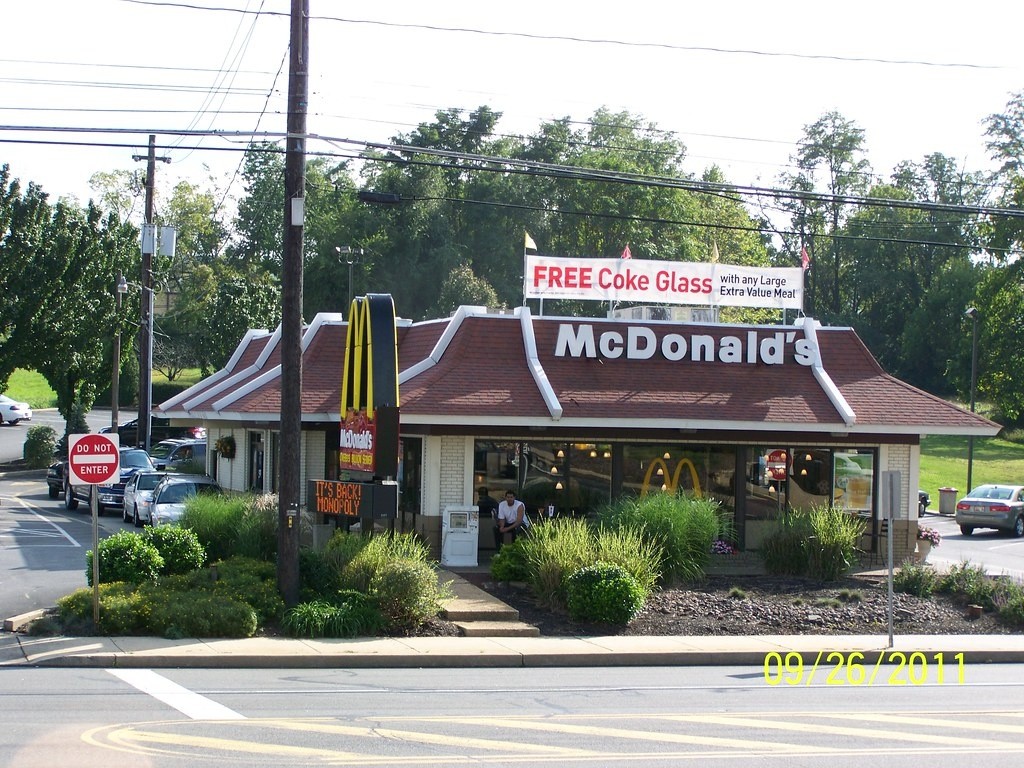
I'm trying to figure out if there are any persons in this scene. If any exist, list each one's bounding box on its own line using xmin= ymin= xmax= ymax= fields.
xmin=494 ymin=490 xmax=531 ymax=552
xmin=477 ymin=487 xmax=499 ymax=518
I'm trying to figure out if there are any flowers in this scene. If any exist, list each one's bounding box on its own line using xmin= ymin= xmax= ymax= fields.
xmin=916 ymin=524 xmax=941 ymax=549
xmin=214 ymin=436 xmax=235 ymax=453
xmin=710 ymin=540 xmax=733 ymax=557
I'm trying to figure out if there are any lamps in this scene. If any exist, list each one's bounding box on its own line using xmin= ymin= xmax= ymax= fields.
xmin=551 ymin=466 xmax=558 ymax=473
xmin=555 ymin=481 xmax=563 ymax=490
xmin=806 ymin=449 xmax=812 ymax=460
xmin=657 ymin=467 xmax=664 ymax=475
xmin=556 ymin=450 xmax=565 ymax=457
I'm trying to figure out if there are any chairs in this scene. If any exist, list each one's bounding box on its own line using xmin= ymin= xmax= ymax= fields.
xmin=861 ymin=518 xmax=894 ymax=569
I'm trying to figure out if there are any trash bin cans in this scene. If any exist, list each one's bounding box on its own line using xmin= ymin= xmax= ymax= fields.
xmin=938 ymin=486 xmax=958 ymax=514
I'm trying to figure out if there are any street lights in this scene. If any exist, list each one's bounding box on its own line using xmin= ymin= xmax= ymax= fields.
xmin=965 ymin=308 xmax=978 ymax=496
xmin=116 ymin=276 xmax=155 ymax=452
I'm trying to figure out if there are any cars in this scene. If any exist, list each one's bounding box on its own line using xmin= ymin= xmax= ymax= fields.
xmin=919 ymin=490 xmax=932 ymax=518
xmin=0 ymin=394 xmax=33 ymax=425
xmin=46 ymin=453 xmax=69 ymax=498
xmin=956 ymin=484 xmax=1024 ymax=538
xmin=148 ymin=437 xmax=206 ymax=474
xmin=145 ymin=472 xmax=228 ymax=531
xmin=62 ymin=447 xmax=165 ymax=516
xmin=122 ymin=469 xmax=185 ymax=527
xmin=97 ymin=412 xmax=206 ymax=452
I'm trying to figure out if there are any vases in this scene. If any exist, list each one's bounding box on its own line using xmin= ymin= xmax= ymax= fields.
xmin=222 ymin=454 xmax=234 ymax=458
xmin=916 ymin=539 xmax=933 ymax=565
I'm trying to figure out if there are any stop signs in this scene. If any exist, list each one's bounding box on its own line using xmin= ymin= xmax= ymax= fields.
xmin=70 ymin=435 xmax=119 ymax=483
xmin=768 ymin=450 xmax=793 ymax=474
